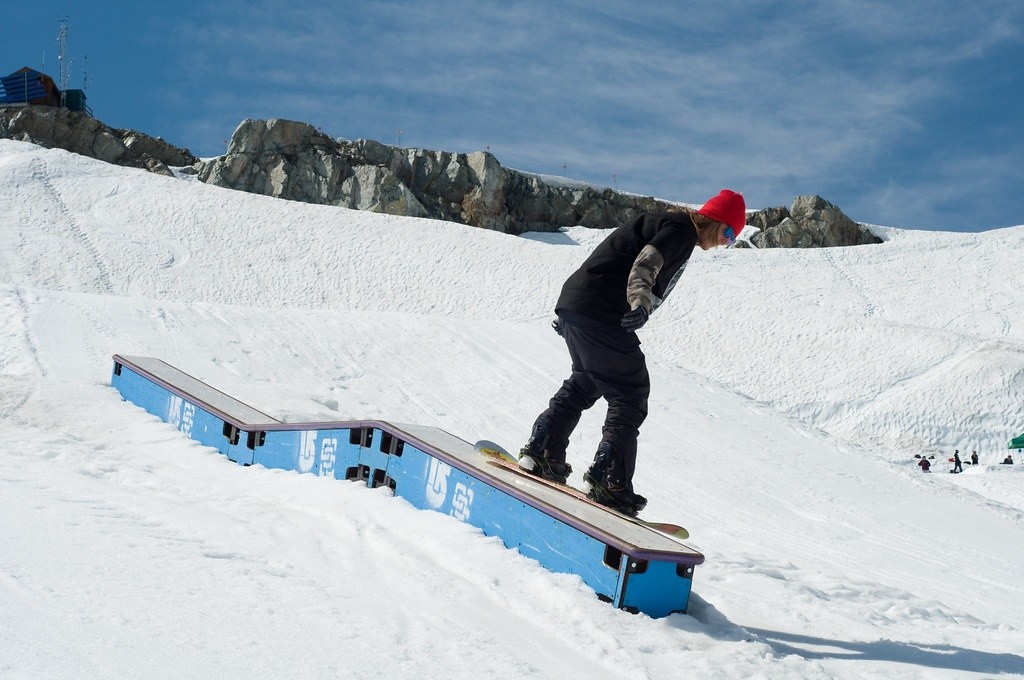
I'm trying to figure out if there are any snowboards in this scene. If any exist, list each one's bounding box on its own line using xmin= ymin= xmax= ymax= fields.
xmin=473 ymin=438 xmax=692 ymax=541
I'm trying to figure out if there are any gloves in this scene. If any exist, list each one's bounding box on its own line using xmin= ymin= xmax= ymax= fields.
xmin=621 ymin=306 xmax=651 ymax=332
xmin=552 ymin=315 xmax=566 ymax=337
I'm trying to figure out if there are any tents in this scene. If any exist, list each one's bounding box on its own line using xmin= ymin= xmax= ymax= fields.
xmin=1007 ymin=433 xmax=1024 ymax=455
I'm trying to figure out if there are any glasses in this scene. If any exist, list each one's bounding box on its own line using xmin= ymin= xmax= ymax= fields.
xmin=723 ymin=222 xmax=736 ymax=241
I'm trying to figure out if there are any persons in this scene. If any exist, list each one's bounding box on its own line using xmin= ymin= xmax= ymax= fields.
xmin=971 ymin=451 xmax=979 ymax=465
xmin=918 ymin=456 xmax=931 ymax=473
xmin=953 ymin=449 xmax=963 ymax=473
xmin=518 ymin=190 xmax=746 ymax=517
xmin=1004 ymin=455 xmax=1013 ymax=464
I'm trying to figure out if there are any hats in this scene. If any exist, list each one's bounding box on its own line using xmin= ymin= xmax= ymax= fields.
xmin=697 ymin=190 xmax=746 ymax=239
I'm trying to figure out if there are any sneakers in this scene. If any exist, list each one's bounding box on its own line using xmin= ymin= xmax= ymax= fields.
xmin=583 ymin=438 xmax=648 ymax=512
xmin=515 ymin=413 xmax=572 ymax=484
